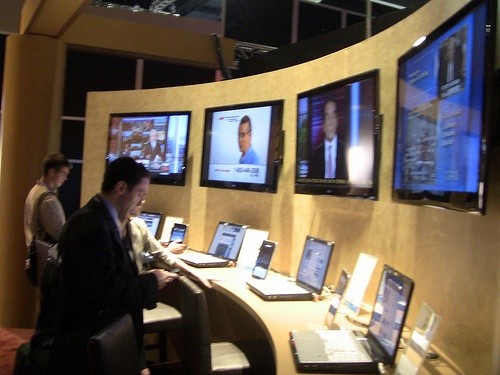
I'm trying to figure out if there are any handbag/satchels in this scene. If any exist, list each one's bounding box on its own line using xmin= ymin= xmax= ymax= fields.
xmin=26 ymin=234 xmax=59 ymax=286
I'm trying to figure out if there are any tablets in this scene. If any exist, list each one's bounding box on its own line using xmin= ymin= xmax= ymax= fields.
xmin=251 ymin=240 xmax=276 ymax=280
xmin=168 ymin=223 xmax=187 ymax=247
xmin=324 ymin=270 xmax=349 ymax=329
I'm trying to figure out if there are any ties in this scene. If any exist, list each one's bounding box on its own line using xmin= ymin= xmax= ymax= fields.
xmin=328 ymin=145 xmax=332 ymax=178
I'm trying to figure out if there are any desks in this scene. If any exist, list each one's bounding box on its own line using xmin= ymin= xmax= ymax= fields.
xmin=140 ymin=244 xmax=465 ymax=375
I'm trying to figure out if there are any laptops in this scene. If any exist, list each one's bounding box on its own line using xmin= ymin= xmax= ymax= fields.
xmin=135 ymin=211 xmax=163 ymax=239
xmin=246 ymin=235 xmax=336 ymax=302
xmin=175 ymin=221 xmax=248 ymax=268
xmin=289 ymin=264 xmax=415 ymax=373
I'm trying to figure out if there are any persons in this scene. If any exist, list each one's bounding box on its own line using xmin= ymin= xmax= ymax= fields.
xmin=57 ymin=156 xmax=179 ymax=375
xmin=238 ymin=116 xmax=259 ymax=164
xmin=23 ymin=152 xmax=73 ymax=325
xmin=438 ymin=36 xmax=464 ymax=95
xmin=144 ymin=129 xmax=161 ymax=161
xmin=306 ymin=98 xmax=348 ymax=179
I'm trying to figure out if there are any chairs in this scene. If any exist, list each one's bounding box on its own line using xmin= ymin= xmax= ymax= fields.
xmin=33 ymin=237 xmax=55 ymax=331
xmin=85 ymin=311 xmax=142 ymax=375
xmin=140 ymin=301 xmax=183 ymax=375
xmin=172 ymin=275 xmax=251 ymax=375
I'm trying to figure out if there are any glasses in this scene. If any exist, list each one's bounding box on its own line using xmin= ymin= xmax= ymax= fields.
xmin=239 ymin=131 xmax=249 ymax=137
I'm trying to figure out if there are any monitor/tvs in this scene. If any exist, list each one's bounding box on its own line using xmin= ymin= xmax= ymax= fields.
xmin=105 ymin=110 xmax=192 ymax=186
xmin=200 ymin=99 xmax=284 ymax=194
xmin=294 ymin=68 xmax=381 ymax=200
xmin=392 ymin=0 xmax=498 ymax=215
